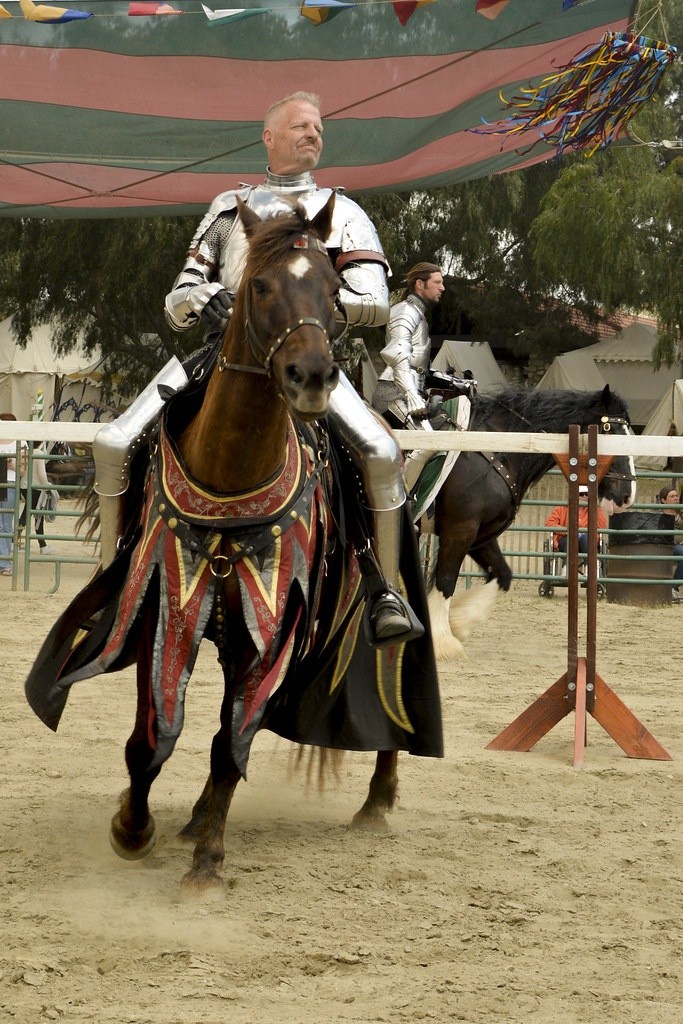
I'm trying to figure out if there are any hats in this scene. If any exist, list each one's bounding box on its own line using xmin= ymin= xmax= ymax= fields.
xmin=579 ymin=485 xmax=588 ymax=492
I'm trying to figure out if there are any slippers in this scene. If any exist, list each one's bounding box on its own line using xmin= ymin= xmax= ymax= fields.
xmin=1 ymin=568 xmax=12 ymax=576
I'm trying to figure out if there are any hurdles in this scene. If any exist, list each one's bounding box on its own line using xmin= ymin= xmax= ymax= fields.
xmin=0 ymin=418 xmax=682 ymax=766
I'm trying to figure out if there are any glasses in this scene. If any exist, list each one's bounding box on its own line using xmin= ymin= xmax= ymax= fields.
xmin=579 ymin=492 xmax=588 ymax=496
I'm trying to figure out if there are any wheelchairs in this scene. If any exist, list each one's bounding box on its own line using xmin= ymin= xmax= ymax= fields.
xmin=537 ymin=531 xmax=605 ymax=601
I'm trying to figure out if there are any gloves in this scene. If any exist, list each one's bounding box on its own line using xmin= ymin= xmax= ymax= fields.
xmin=393 ymin=369 xmax=429 ymax=422
xmin=424 ymin=367 xmax=478 ymax=394
xmin=164 ymin=280 xmax=233 ymax=328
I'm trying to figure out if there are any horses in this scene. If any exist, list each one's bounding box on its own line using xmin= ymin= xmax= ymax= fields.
xmin=416 ymin=382 xmax=637 ymax=599
xmin=106 ymin=194 xmax=401 ymax=880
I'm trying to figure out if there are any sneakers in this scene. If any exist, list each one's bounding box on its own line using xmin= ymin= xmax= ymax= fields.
xmin=672 ymin=587 xmax=681 ymax=599
xmin=40 ymin=543 xmax=57 ymax=555
xmin=561 ymin=565 xmax=566 ymax=577
xmin=373 ymin=598 xmax=412 ymax=639
xmin=583 ymin=564 xmax=587 ymax=576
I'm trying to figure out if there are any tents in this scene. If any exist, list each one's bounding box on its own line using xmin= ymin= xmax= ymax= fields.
xmin=529 ymin=353 xmax=607 ymax=393
xmin=355 ymin=338 xmax=379 ymax=404
xmin=0 ymin=309 xmax=168 ymax=424
xmin=563 ymin=322 xmax=683 ymax=425
xmin=431 ymin=340 xmax=510 ymax=395
xmin=633 ymin=379 xmax=683 ymax=471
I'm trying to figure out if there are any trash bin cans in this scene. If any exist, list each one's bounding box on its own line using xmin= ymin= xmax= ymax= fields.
xmin=608 ymin=512 xmax=675 ymax=609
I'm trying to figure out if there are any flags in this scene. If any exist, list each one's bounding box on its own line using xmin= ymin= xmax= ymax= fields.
xmin=20 ymin=0 xmax=94 ymax=24
xmin=0 ymin=4 xmax=11 ymax=19
xmin=201 ymin=2 xmax=272 ymax=28
xmin=392 ymin=0 xmax=437 ymax=27
xmin=127 ymin=2 xmax=183 ymax=15
xmin=475 ymin=0 xmax=511 ymax=20
xmin=300 ymin=0 xmax=356 ymax=26
xmin=562 ymin=0 xmax=593 ymax=11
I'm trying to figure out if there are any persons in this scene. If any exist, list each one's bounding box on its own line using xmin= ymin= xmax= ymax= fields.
xmin=90 ymin=92 xmax=415 ymax=638
xmin=372 ymin=262 xmax=477 ymax=496
xmin=0 ymin=413 xmax=52 ymax=575
xmin=545 ymin=485 xmax=608 ymax=577
xmin=657 ymin=487 xmax=683 ymax=598
